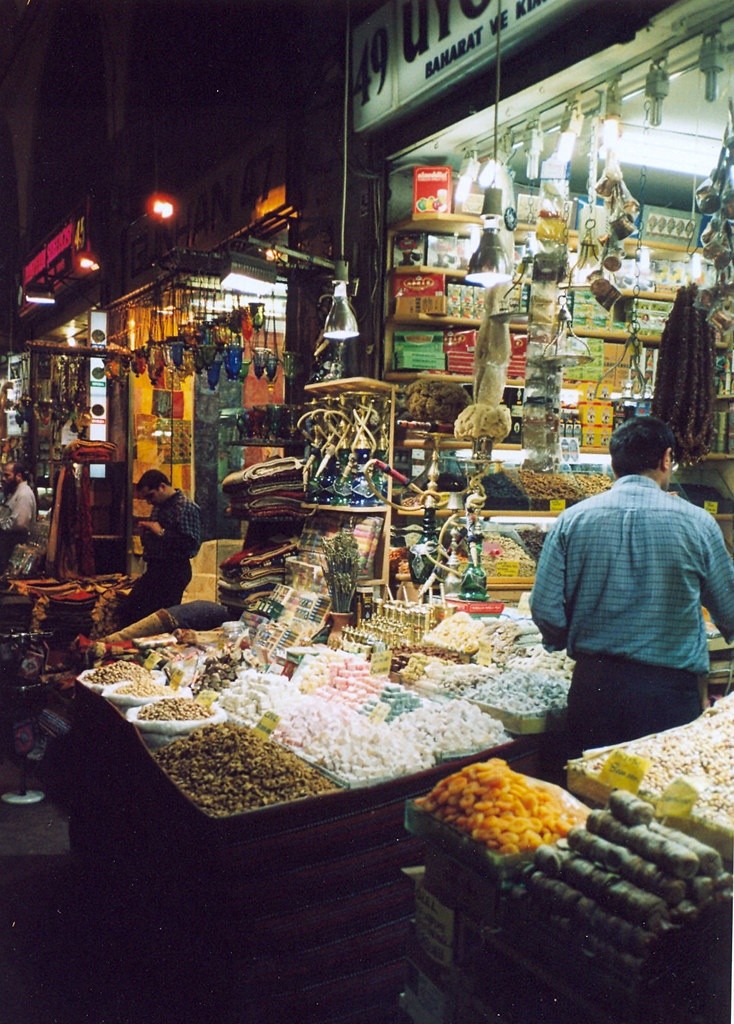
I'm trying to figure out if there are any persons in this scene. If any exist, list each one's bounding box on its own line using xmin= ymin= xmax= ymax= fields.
xmin=118 ymin=469 xmax=202 ymax=631
xmin=529 ymin=417 xmax=734 ymax=791
xmin=0 ymin=460 xmax=37 ymax=577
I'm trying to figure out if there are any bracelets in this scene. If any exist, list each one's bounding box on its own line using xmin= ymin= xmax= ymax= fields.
xmin=157 ymin=528 xmax=165 ymax=537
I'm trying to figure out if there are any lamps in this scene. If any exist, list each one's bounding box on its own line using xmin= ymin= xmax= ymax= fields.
xmin=464 ymin=0 xmax=514 ymax=288
xmin=220 ymin=238 xmax=276 ymax=295
xmin=25 ymin=281 xmax=55 ymax=304
xmin=323 ymin=0 xmax=360 ymax=339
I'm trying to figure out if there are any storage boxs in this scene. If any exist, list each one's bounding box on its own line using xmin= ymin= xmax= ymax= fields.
xmin=392 ymin=274 xmax=675 ymax=336
xmin=392 ymin=330 xmax=659 ymax=400
xmin=463 ymin=385 xmax=635 ymax=448
xmin=413 ymin=165 xmax=702 ymax=247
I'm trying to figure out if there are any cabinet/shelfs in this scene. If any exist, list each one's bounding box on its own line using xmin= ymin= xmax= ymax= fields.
xmin=219 ymin=439 xmax=310 ymax=609
xmin=383 ymin=212 xmax=734 ymax=601
xmin=300 ymin=377 xmax=395 ymax=602
xmin=54 ymin=459 xmax=129 ymax=539
